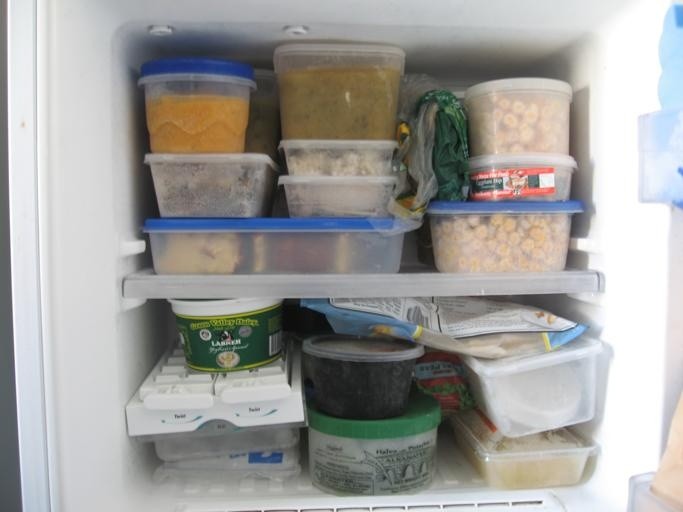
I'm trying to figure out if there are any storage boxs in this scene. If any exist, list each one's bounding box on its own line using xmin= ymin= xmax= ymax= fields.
xmin=140 ymin=216 xmax=409 ymax=274
